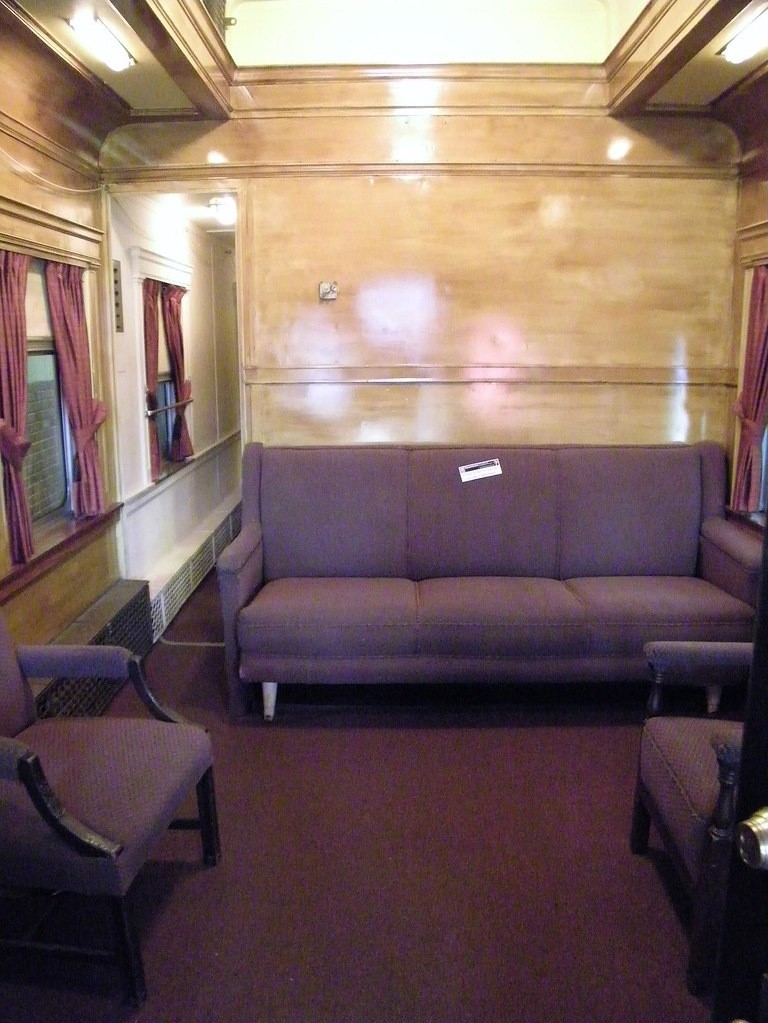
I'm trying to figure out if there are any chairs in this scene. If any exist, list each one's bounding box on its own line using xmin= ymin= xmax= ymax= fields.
xmin=629 ymin=642 xmax=756 ymax=993
xmin=0 ymin=608 xmax=218 ymax=1000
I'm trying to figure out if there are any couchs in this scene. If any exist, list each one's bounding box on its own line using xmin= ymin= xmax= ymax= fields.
xmin=213 ymin=443 xmax=768 ymax=721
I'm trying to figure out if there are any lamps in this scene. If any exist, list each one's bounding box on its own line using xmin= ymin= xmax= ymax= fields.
xmin=721 ymin=7 xmax=768 ymax=64
xmin=62 ymin=15 xmax=138 ymax=71
